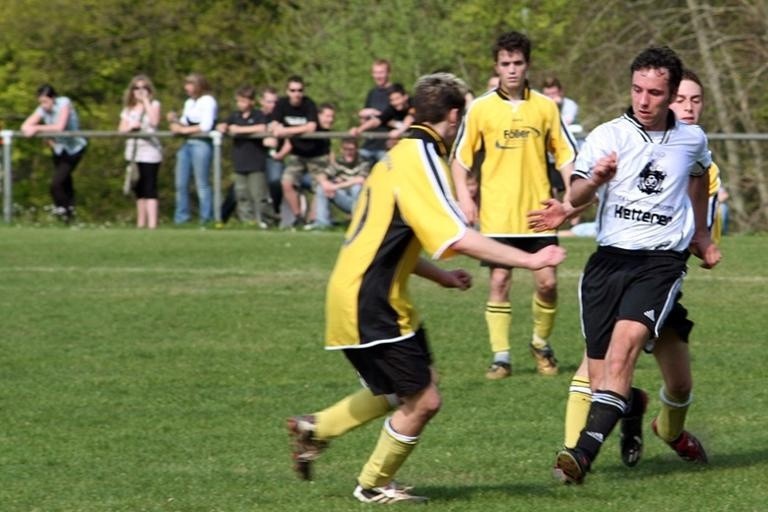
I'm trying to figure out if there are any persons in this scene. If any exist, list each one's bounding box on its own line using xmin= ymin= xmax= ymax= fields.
xmin=449 ymin=32 xmax=579 ymax=379
xmin=286 ymin=72 xmax=567 ymax=507
xmin=21 ymin=84 xmax=86 ymax=228
xmin=118 ymin=74 xmax=161 ymax=230
xmin=527 ymin=73 xmax=720 ymax=481
xmin=557 ymin=46 xmax=721 ymax=487
xmin=166 ymin=74 xmax=218 ymax=229
xmin=218 ymin=61 xmax=582 ymax=232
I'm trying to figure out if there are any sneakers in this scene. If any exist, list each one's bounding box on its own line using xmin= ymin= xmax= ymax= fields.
xmin=353 ymin=480 xmax=428 ymax=505
xmin=484 ymin=360 xmax=511 ymax=380
xmin=528 ymin=339 xmax=560 ymax=376
xmin=286 ymin=414 xmax=328 ymax=484
xmin=555 ymin=387 xmax=707 ymax=486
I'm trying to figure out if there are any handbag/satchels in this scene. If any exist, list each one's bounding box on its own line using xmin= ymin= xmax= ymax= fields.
xmin=130 ymin=162 xmax=140 ymax=186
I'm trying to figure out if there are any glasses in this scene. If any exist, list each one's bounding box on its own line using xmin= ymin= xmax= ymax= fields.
xmin=289 ymin=87 xmax=304 ymax=94
